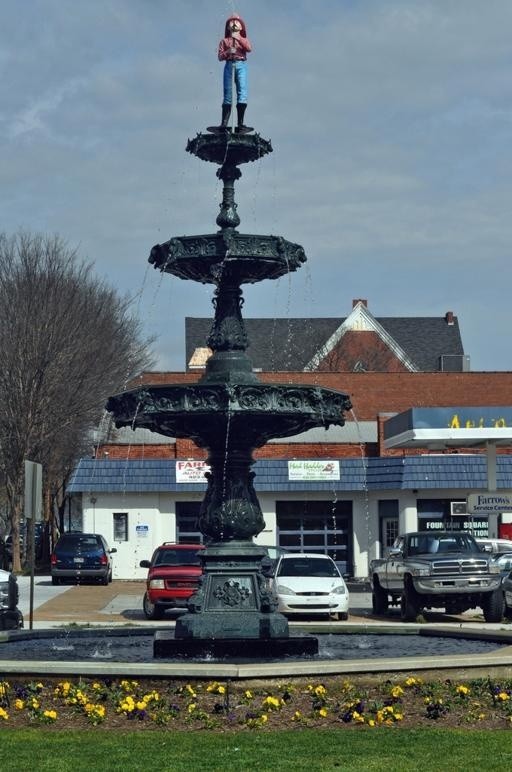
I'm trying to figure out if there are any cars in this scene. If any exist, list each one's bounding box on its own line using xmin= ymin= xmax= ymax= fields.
xmin=0 ymin=521 xmax=44 ymax=630
xmin=475 ymin=531 xmax=512 ymax=617
xmin=265 ymin=553 xmax=349 ymax=621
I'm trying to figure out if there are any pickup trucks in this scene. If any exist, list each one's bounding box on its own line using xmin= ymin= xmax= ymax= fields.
xmin=369 ymin=531 xmax=502 ymax=622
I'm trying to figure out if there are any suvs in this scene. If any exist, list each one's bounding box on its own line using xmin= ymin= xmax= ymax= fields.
xmin=140 ymin=541 xmax=206 ymax=620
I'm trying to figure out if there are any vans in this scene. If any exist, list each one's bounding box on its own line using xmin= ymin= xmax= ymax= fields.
xmin=51 ymin=533 xmax=117 ymax=585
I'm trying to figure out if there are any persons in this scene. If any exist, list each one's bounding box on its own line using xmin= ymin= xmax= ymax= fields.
xmin=217 ymin=15 xmax=255 ymax=134
